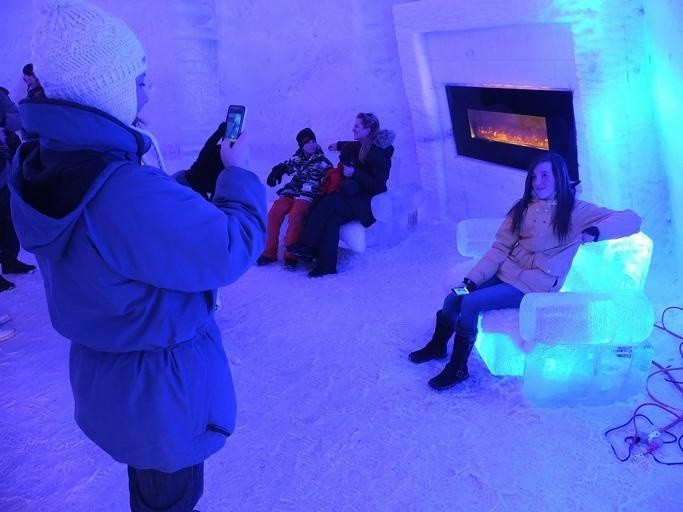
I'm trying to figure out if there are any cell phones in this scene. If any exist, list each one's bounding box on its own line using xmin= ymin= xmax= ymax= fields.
xmin=217 ymin=104 xmax=248 ymax=169
xmin=451 ymin=287 xmax=470 ymax=296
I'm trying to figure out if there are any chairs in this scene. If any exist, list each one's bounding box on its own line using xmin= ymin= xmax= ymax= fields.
xmin=444 ymin=215 xmax=654 ymax=409
xmin=337 ymin=158 xmax=422 ymax=256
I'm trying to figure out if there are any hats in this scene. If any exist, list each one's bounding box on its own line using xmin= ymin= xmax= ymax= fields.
xmin=30 ymin=0 xmax=147 ymax=125
xmin=296 ymin=128 xmax=317 ymax=150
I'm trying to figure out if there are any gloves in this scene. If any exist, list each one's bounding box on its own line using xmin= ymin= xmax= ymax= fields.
xmin=185 ymin=123 xmax=231 ymax=194
xmin=266 ymin=162 xmax=288 ymax=186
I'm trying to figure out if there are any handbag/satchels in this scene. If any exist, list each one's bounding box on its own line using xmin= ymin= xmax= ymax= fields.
xmin=320 ymin=162 xmax=342 ymax=197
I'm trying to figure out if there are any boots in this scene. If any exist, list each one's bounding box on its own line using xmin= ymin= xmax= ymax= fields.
xmin=427 ymin=328 xmax=477 ymax=390
xmin=408 ymin=308 xmax=456 ymax=364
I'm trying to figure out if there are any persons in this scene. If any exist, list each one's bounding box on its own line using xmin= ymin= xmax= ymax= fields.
xmin=408 ymin=154 xmax=641 ymax=391
xmin=0 ymin=61 xmax=45 ymax=342
xmin=255 ymin=112 xmax=395 ymax=277
xmin=11 ymin=4 xmax=268 ymax=512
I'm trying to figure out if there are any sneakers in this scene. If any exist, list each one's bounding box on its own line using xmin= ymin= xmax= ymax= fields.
xmin=256 ymin=240 xmax=338 ymax=278
xmin=1 ymin=259 xmax=37 ymax=293
